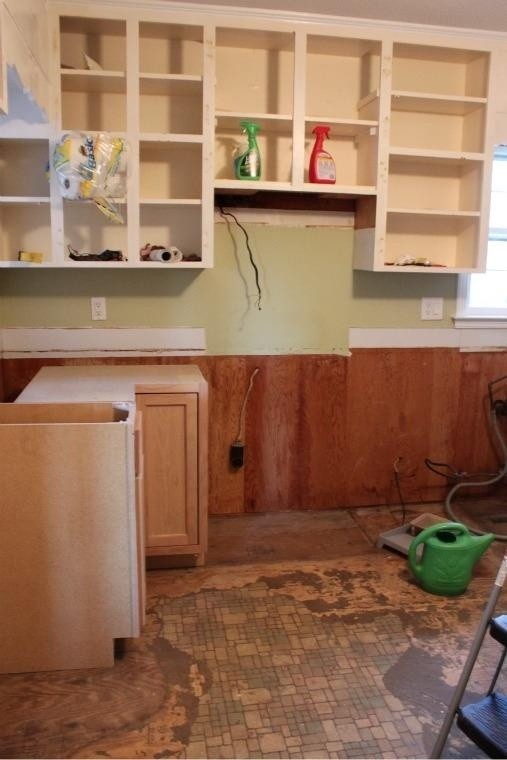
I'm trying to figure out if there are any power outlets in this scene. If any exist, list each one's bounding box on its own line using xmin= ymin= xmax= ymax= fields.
xmin=88 ymin=297 xmax=106 ymax=321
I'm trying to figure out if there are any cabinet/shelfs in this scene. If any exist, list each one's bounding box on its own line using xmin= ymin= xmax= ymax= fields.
xmin=8 ymin=362 xmax=218 ymax=577
xmin=0 ymin=3 xmax=506 ymax=277
xmin=0 ymin=399 xmax=146 ymax=683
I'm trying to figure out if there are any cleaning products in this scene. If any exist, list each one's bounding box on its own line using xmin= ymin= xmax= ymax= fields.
xmin=310 ymin=125 xmax=338 ymax=184
xmin=234 ymin=120 xmax=261 ymax=180
xmin=148 ymin=247 xmax=173 ymax=261
